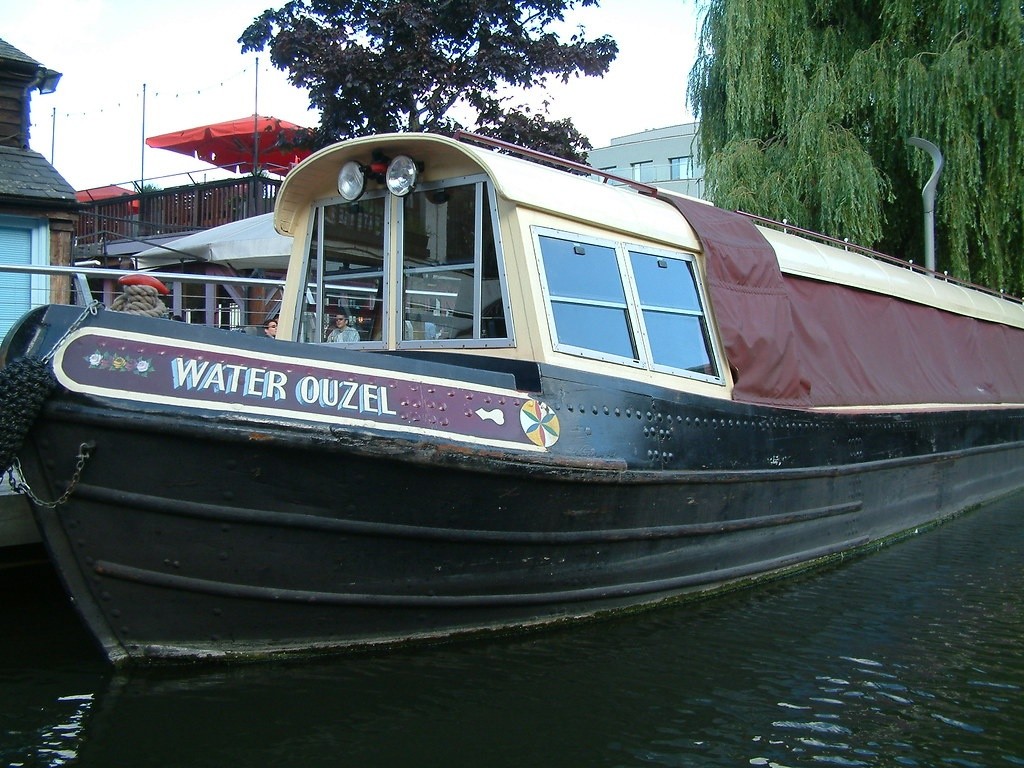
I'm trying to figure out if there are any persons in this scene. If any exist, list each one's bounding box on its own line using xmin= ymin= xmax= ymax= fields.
xmin=425 ymin=323 xmax=436 ymax=340
xmin=263 ymin=320 xmax=278 ymax=339
xmin=326 ymin=311 xmax=360 ymax=343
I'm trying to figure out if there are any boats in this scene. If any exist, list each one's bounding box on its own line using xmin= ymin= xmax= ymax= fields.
xmin=0 ymin=127 xmax=1024 ymax=675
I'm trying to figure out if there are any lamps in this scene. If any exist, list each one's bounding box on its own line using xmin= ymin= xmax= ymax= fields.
xmin=38 ymin=69 xmax=62 ymax=95
xmin=336 ymin=148 xmax=426 ymax=202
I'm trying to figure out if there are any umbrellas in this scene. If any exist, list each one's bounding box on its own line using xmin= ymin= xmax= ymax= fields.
xmin=76 ymin=184 xmax=139 ymax=216
xmin=145 ymin=113 xmax=317 ymax=178
